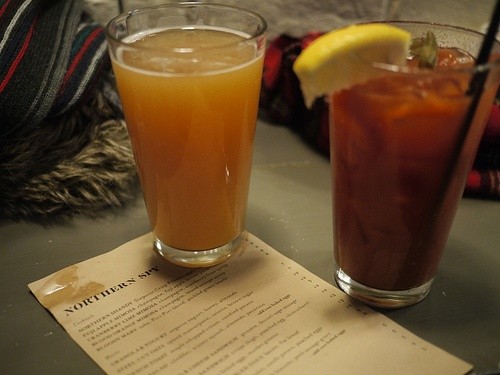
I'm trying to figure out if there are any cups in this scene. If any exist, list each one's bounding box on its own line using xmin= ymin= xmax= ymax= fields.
xmin=329 ymin=22 xmax=499 ymax=309
xmin=106 ymin=3 xmax=269 ymax=268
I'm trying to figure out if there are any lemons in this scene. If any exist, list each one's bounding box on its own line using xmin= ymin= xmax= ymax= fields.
xmin=293 ymin=23 xmax=412 ymax=107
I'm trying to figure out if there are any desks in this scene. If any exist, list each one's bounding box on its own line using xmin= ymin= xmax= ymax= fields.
xmin=0 ymin=117 xmax=500 ymax=375
